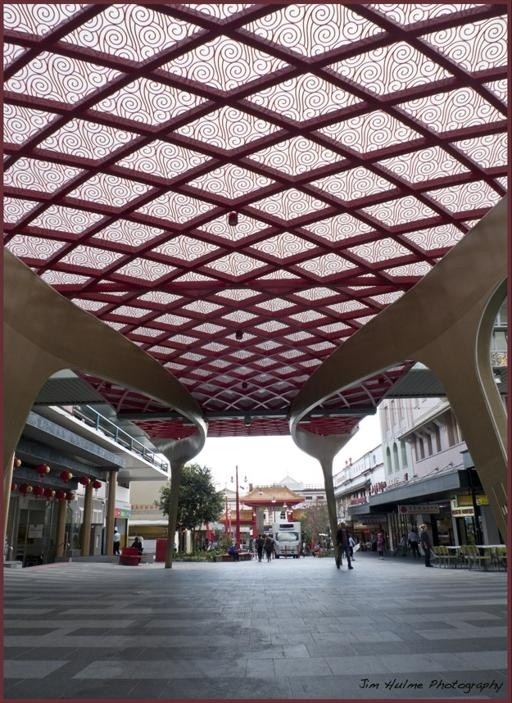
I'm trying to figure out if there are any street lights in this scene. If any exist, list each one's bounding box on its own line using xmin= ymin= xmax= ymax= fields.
xmin=227 ymin=509 xmax=232 ymax=539
xmin=460 ymin=449 xmax=488 ymax=571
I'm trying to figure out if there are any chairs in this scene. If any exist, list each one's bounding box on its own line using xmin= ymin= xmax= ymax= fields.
xmin=427 ymin=542 xmax=508 ymax=573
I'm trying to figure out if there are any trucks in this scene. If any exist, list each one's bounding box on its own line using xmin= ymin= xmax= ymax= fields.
xmin=273 ymin=521 xmax=301 ymax=559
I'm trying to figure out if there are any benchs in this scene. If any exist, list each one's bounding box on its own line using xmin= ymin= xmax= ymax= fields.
xmin=212 ymin=547 xmax=253 ymax=562
xmin=115 ymin=545 xmax=149 ymax=565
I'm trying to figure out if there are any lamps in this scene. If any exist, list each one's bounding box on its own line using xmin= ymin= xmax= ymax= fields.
xmin=224 ymin=209 xmax=252 ymax=428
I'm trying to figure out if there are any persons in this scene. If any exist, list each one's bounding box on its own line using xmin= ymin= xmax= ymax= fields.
xmin=417 ymin=522 xmax=433 ymax=567
xmin=114 ymin=525 xmax=419 ymax=561
xmin=336 ymin=521 xmax=354 ymax=569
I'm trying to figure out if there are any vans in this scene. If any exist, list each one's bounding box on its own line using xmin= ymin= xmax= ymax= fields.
xmin=318 ymin=532 xmax=331 ymax=547
